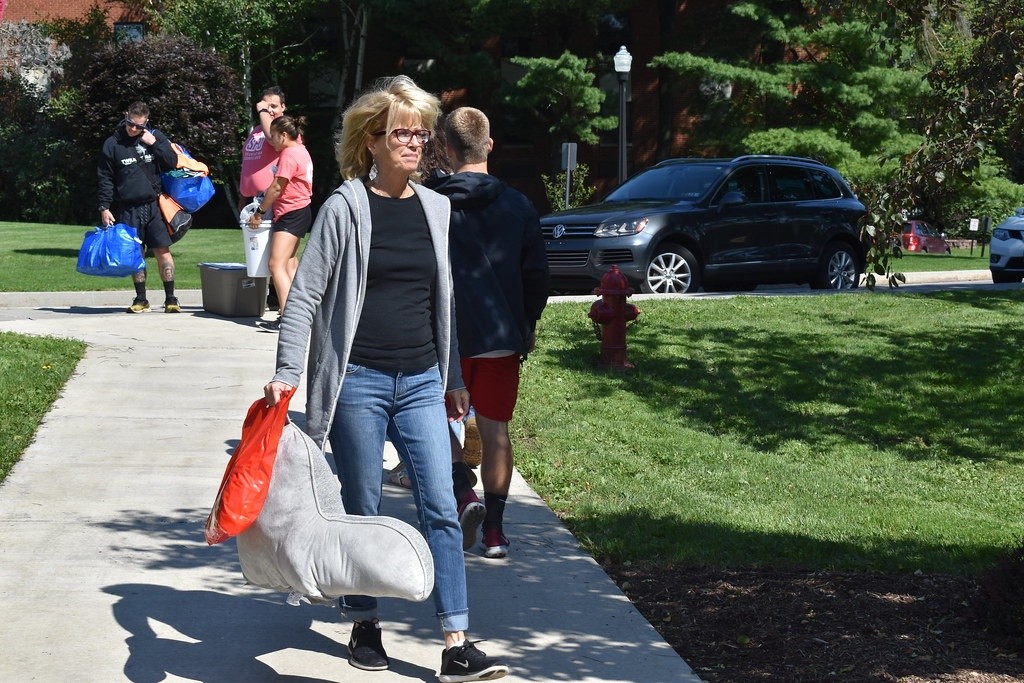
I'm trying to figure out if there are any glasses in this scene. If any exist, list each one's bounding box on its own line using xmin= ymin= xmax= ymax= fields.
xmin=125 ymin=113 xmax=148 ymax=130
xmin=372 ymin=127 xmax=431 ymax=144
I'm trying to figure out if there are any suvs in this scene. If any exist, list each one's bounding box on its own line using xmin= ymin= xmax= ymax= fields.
xmin=541 ymin=154 xmax=877 ymax=295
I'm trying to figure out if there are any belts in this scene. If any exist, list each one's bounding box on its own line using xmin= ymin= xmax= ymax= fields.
xmin=256 ymin=191 xmax=264 ymax=197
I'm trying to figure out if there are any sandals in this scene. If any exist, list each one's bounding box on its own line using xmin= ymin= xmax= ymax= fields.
xmin=389 ymin=471 xmax=413 ymax=488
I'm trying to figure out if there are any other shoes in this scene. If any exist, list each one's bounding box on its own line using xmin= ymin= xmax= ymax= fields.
xmin=264 ymin=293 xmax=281 ymax=310
xmin=463 ymin=463 xmax=478 ymax=486
xmin=464 ymin=415 xmax=485 ymax=467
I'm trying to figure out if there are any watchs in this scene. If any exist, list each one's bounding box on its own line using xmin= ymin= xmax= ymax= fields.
xmin=256 ymin=207 xmax=266 ymax=215
xmin=99 ymin=206 xmax=107 ymax=212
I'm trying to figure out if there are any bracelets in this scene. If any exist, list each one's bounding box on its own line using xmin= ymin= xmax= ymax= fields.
xmin=259 ymin=109 xmax=268 ymax=113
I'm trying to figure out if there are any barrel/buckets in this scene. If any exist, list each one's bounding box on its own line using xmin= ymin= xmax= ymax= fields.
xmin=239 ymin=220 xmax=274 ymax=277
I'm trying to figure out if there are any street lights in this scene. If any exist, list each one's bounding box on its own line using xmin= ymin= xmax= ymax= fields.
xmin=614 ymin=45 xmax=633 ymax=186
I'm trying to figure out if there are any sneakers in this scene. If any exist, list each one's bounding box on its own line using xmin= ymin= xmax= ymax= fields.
xmin=458 ymin=488 xmax=486 ymax=550
xmin=127 ymin=298 xmax=152 ymax=312
xmin=258 ymin=316 xmax=282 ymax=332
xmin=163 ymin=296 xmax=182 ymax=312
xmin=480 ymin=526 xmax=511 ymax=558
xmin=437 ymin=637 xmax=509 ymax=683
xmin=347 ymin=617 xmax=390 ymax=670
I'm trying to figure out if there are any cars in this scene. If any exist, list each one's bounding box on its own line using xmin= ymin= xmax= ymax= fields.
xmin=900 ymin=220 xmax=950 ymax=255
xmin=989 ymin=207 xmax=1024 ymax=283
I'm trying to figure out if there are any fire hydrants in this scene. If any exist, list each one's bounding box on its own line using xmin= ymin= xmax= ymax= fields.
xmin=588 ymin=264 xmax=642 ymax=370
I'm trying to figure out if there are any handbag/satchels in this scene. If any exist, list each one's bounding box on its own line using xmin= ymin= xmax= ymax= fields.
xmin=239 ymin=199 xmax=274 ymax=221
xmin=76 ymin=222 xmax=146 ymax=277
xmin=147 ymin=129 xmax=216 ymax=243
xmin=206 ymin=386 xmax=296 ymax=543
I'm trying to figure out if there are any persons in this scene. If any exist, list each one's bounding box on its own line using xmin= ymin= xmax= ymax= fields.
xmin=423 ymin=106 xmax=551 ymax=557
xmin=367 ymin=117 xmax=484 ymax=487
xmin=265 ymin=75 xmax=510 ymax=683
xmin=97 ymin=100 xmax=180 ymax=314
xmin=240 ymin=87 xmax=303 ymax=311
xmin=247 ymin=115 xmax=312 ymax=334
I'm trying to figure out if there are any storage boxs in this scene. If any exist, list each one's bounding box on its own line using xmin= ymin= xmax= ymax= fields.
xmin=198 ymin=262 xmax=268 ymax=318
